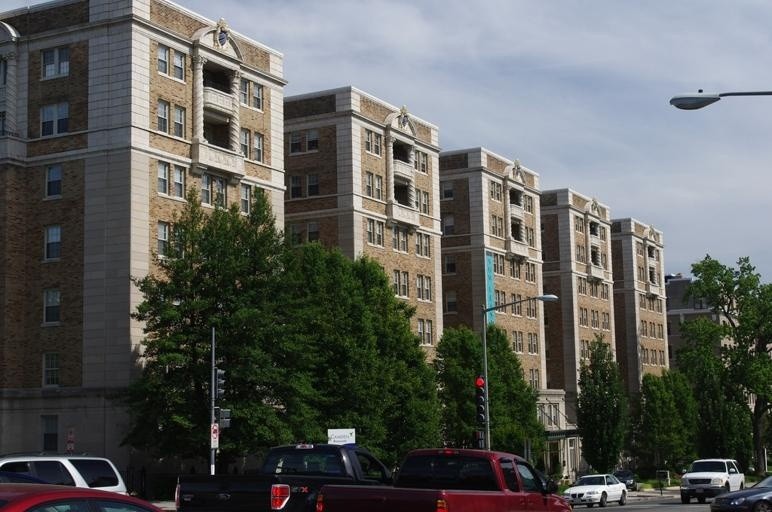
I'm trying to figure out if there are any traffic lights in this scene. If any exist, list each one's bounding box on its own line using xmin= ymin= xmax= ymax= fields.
xmin=473 ymin=372 xmax=486 ymax=418
xmin=213 ymin=366 xmax=226 ymax=400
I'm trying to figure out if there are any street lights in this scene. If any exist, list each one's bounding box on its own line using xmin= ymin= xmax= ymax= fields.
xmin=481 ymin=292 xmax=559 ymax=450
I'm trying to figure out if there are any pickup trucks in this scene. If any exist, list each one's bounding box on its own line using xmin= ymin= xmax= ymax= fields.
xmin=173 ymin=441 xmax=393 ymax=512
xmin=313 ymin=447 xmax=572 ymax=512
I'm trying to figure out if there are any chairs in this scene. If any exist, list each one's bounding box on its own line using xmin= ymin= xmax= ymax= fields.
xmin=324 ymin=457 xmax=339 ymax=471
xmin=287 ymin=455 xmax=309 ymax=474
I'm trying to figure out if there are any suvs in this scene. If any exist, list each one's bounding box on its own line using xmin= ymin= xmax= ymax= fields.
xmin=679 ymin=457 xmax=745 ymax=502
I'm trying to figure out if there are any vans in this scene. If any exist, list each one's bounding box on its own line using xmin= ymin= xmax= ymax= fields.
xmin=0 ymin=455 xmax=129 ymax=512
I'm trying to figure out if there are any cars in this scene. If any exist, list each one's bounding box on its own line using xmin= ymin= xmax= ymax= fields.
xmin=563 ymin=472 xmax=627 ymax=506
xmin=711 ymin=476 xmax=772 ymax=512
xmin=614 ymin=469 xmax=638 ymax=490
xmin=0 ymin=480 xmax=165 ymax=512
